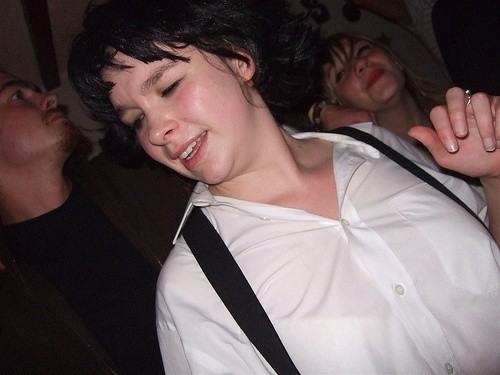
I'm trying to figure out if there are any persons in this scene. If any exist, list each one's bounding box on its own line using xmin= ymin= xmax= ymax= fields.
xmin=0 ymin=68 xmax=382 ymax=375
xmin=311 ymin=31 xmax=449 ymax=148
xmin=66 ymin=0 xmax=500 ymax=375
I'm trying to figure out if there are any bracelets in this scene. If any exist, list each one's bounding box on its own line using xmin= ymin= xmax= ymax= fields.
xmin=312 ymin=97 xmax=342 ymax=132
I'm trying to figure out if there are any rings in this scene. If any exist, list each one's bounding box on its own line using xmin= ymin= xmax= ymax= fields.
xmin=462 ymin=88 xmax=472 ymax=107
